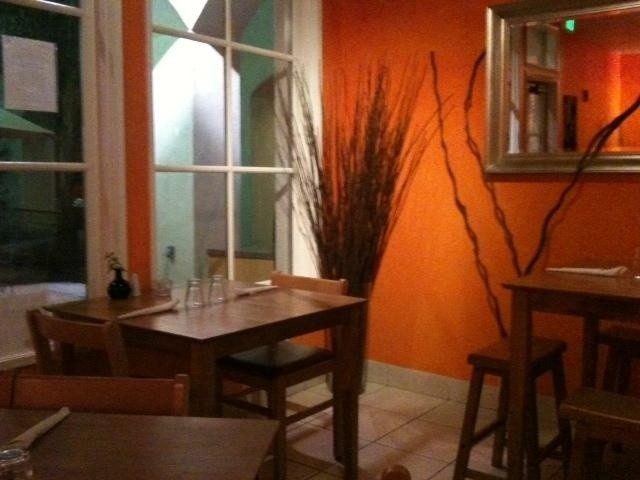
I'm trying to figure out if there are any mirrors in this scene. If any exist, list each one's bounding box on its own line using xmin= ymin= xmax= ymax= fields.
xmin=478 ymin=4 xmax=638 ymax=173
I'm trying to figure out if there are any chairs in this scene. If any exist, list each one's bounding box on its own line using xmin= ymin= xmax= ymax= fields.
xmin=381 ymin=463 xmax=411 ymax=480
xmin=11 ymin=368 xmax=187 ymax=413
xmin=217 ymin=268 xmax=348 ymax=479
xmin=24 ymin=303 xmax=128 ymax=376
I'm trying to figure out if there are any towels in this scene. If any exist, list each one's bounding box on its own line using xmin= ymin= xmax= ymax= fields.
xmin=9 ymin=408 xmax=71 ymax=448
xmin=546 ymin=266 xmax=629 ymax=277
xmin=117 ymin=297 xmax=179 ymax=321
xmin=234 ymin=283 xmax=278 ymax=296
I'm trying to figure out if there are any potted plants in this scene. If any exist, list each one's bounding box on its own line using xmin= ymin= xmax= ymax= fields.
xmin=270 ymin=48 xmax=456 ymax=397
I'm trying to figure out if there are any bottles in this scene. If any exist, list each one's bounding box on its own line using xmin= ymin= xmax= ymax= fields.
xmin=130 ymin=271 xmax=141 ymax=298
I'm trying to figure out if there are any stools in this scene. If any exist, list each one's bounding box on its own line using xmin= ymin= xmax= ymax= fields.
xmin=452 ymin=332 xmax=571 ymax=478
xmin=599 ymin=325 xmax=639 ymax=392
xmin=559 ymin=383 xmax=640 ymax=479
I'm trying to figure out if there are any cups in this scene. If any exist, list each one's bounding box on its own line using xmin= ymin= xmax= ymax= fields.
xmin=185 ymin=279 xmax=204 ymax=308
xmin=0 ymin=445 xmax=33 ymax=480
xmin=152 ymin=278 xmax=172 ymax=297
xmin=209 ymin=275 xmax=226 ymax=301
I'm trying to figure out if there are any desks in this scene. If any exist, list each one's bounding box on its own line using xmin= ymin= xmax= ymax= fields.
xmin=0 ymin=411 xmax=284 ymax=479
xmin=43 ymin=275 xmax=367 ymax=479
xmin=500 ymin=266 xmax=640 ymax=480
